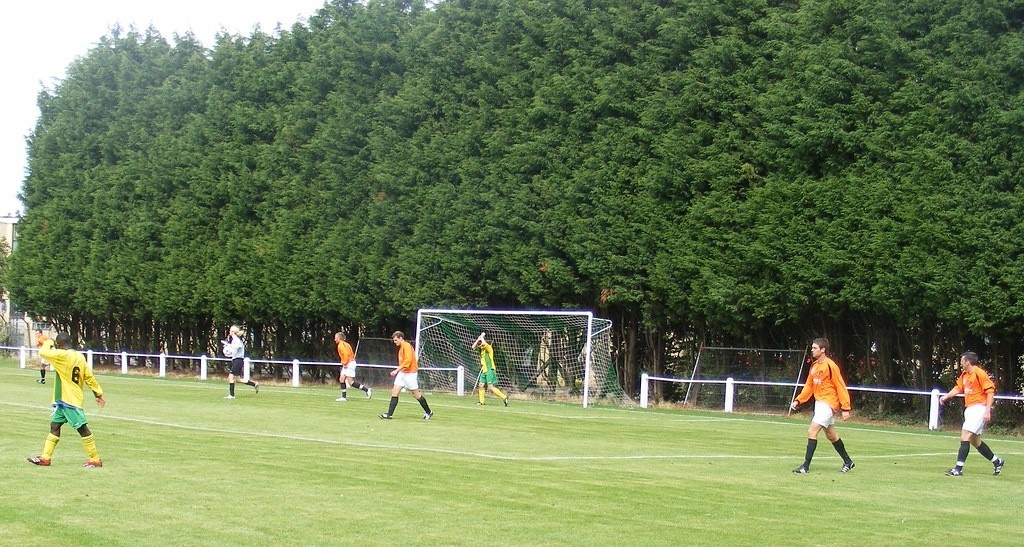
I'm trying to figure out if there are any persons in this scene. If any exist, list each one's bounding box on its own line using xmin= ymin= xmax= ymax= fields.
xmin=221 ymin=325 xmax=259 ymax=398
xmin=791 ymin=337 xmax=855 ymax=473
xmin=334 ymin=332 xmax=372 ymax=401
xmin=939 ymin=351 xmax=1006 ymax=476
xmin=35 ymin=329 xmax=55 ymax=384
xmin=472 ymin=332 xmax=509 ymax=407
xmin=378 ymin=331 xmax=433 ymax=420
xmin=26 ymin=332 xmax=106 ymax=467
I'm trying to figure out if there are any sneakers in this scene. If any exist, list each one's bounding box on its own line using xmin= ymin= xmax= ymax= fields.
xmin=83 ymin=459 xmax=102 ymax=468
xmin=841 ymin=460 xmax=856 ymax=473
xmin=792 ymin=466 xmax=809 ymax=473
xmin=26 ymin=456 xmax=51 ymax=466
xmin=993 ymin=458 xmax=1005 ymax=476
xmin=503 ymin=396 xmax=509 ymax=406
xmin=423 ymin=410 xmax=433 ymax=419
xmin=378 ymin=413 xmax=393 ymax=419
xmin=476 ymin=402 xmax=485 ymax=405
xmin=945 ymin=468 xmax=963 ymax=476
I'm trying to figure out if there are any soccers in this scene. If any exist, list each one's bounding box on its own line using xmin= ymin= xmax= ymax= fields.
xmin=223 ymin=346 xmax=232 ymax=355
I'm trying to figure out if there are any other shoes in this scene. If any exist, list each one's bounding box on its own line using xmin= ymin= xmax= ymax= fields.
xmin=366 ymin=388 xmax=372 ymax=399
xmin=335 ymin=397 xmax=347 ymax=401
xmin=254 ymin=382 xmax=259 ymax=393
xmin=37 ymin=379 xmax=45 ymax=384
xmin=225 ymin=395 xmax=235 ymax=399
